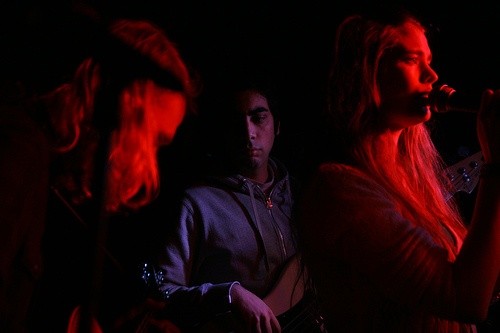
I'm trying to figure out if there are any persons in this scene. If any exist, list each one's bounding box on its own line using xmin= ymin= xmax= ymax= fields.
xmin=299 ymin=10 xmax=500 ymax=333
xmin=0 ymin=20 xmax=196 ymax=333
xmin=158 ymin=90 xmax=308 ymax=333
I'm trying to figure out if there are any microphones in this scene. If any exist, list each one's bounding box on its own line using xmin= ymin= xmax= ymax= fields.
xmin=429 ymin=84 xmax=482 ymax=112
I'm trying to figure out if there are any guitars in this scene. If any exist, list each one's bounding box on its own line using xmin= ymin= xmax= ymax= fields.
xmin=248 ymin=150 xmax=484 ymax=333
